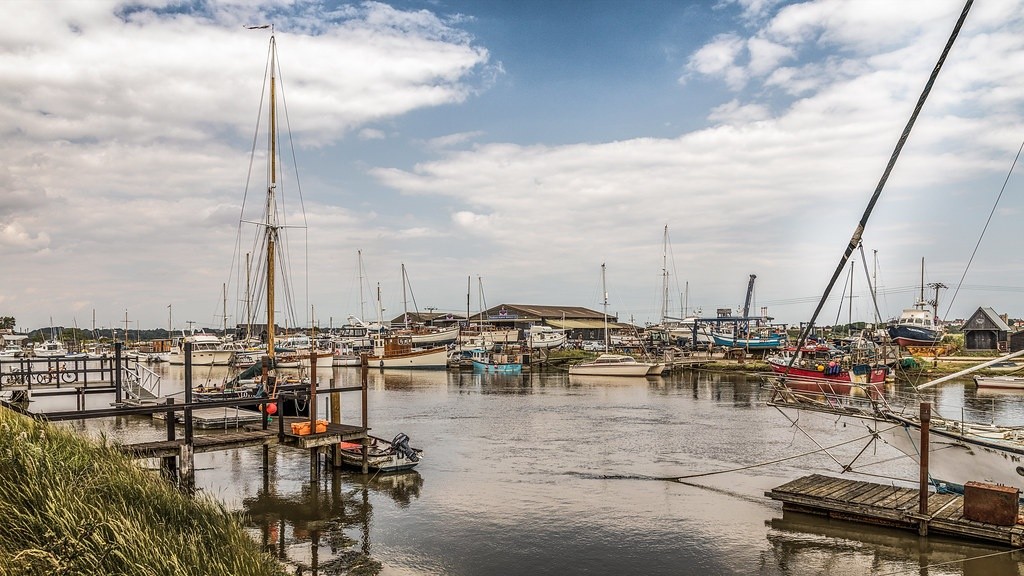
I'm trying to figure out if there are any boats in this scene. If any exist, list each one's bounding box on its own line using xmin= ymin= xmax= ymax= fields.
xmin=339 ymin=432 xmax=427 ymax=475
xmin=852 ymin=391 xmax=1024 ymax=497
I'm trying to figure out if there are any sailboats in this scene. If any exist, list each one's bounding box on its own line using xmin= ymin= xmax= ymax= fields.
xmin=19 ymin=59 xmax=573 ymax=415
xmin=659 ymin=222 xmax=950 ymax=391
xmin=567 ymin=263 xmax=675 ymax=381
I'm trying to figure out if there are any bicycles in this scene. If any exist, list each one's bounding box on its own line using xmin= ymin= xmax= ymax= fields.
xmin=2 ymin=360 xmax=78 ymax=386
